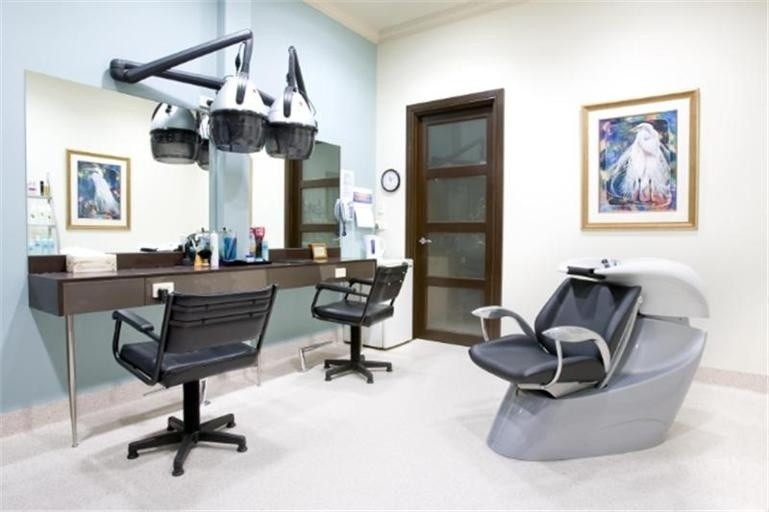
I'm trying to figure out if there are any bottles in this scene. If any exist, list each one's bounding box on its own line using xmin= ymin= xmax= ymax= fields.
xmin=208 ymin=229 xmax=220 ymax=269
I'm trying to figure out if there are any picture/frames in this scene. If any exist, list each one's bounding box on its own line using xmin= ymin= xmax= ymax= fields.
xmin=580 ymin=89 xmax=702 ymax=231
xmin=64 ymin=148 xmax=132 ymax=229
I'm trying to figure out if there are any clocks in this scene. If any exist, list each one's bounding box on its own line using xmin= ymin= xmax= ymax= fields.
xmin=381 ymin=168 xmax=400 ymax=192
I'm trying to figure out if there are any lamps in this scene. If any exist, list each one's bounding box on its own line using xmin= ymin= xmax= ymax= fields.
xmin=149 ymin=100 xmax=210 ymax=170
xmin=111 ymin=30 xmax=318 ymax=161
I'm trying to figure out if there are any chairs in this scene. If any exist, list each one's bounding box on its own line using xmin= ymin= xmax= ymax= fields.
xmin=467 ymin=257 xmax=709 ymax=461
xmin=314 ymin=261 xmax=410 ymax=383
xmin=110 ymin=283 xmax=282 ymax=476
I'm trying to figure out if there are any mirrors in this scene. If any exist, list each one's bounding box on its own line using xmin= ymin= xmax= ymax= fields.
xmin=21 ymin=68 xmax=340 ymax=269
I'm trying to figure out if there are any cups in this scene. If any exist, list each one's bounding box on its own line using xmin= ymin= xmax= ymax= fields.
xmin=223 ymin=231 xmax=236 ymax=262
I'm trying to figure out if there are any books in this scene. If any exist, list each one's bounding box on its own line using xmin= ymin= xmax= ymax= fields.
xmin=140 ymin=246 xmax=160 ymax=252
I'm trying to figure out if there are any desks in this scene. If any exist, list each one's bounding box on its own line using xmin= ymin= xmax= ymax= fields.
xmin=26 ymin=259 xmax=350 ymax=452
